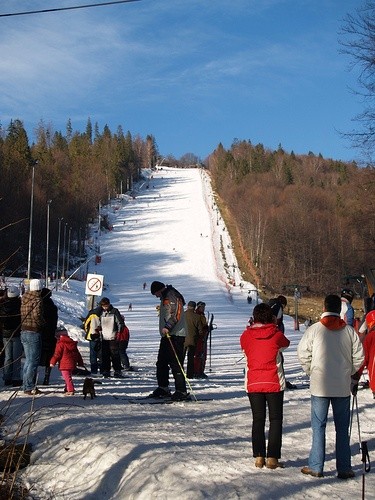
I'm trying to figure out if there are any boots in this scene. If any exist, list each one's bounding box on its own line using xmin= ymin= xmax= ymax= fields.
xmin=43 ymin=367 xmax=51 ymax=386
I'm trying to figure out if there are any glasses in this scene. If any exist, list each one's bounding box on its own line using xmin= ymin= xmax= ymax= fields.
xmin=188 ymin=306 xmax=195 ymax=310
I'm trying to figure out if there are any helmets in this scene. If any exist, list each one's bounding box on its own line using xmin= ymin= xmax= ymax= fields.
xmin=341 ymin=289 xmax=355 ymax=304
xmin=365 ymin=310 xmax=375 ymax=330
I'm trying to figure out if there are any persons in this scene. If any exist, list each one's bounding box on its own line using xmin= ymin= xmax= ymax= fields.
xmin=146 ymin=281 xmax=191 ymax=400
xmin=34 ymin=288 xmax=58 ymax=385
xmin=50 ymin=324 xmax=84 ymax=396
xmin=84 ymin=303 xmax=105 ymax=373
xmin=183 ymin=301 xmax=205 ymax=379
xmin=95 ymin=296 xmax=124 ymax=378
xmin=116 ymin=314 xmax=133 ymax=370
xmin=20 ymin=279 xmax=44 ymax=394
xmin=0 ymin=285 xmax=25 ymax=386
xmin=193 ymin=301 xmax=213 ymax=379
xmin=240 ymin=303 xmax=290 ymax=469
xmin=296 ymin=295 xmax=366 ymax=479
xmin=339 ymin=288 xmax=354 ymax=327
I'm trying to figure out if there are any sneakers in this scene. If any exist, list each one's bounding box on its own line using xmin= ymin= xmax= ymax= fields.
xmin=123 ymin=364 xmax=130 ymax=371
xmin=66 ymin=391 xmax=74 ymax=395
xmin=4 ymin=380 xmax=10 ymax=386
xmin=25 ymin=390 xmax=35 ymax=395
xmin=286 ymin=382 xmax=298 ymax=389
xmin=255 ymin=456 xmax=265 ymax=467
xmin=171 ymin=391 xmax=187 ymax=399
xmin=200 ymin=372 xmax=208 ymax=378
xmin=153 ymin=387 xmax=171 ymax=397
xmin=31 ymin=390 xmax=42 ymax=394
xmin=337 ymin=470 xmax=356 ymax=479
xmin=102 ymin=370 xmax=110 ymax=376
xmin=301 ymin=467 xmax=323 ymax=478
xmin=12 ymin=379 xmax=22 ymax=387
xmin=266 ymin=458 xmax=284 ymax=468
xmin=114 ymin=370 xmax=122 ymax=377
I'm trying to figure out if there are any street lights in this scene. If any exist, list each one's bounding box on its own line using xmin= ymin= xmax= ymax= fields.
xmin=60 ymin=223 xmax=68 ymax=285
xmin=27 ymin=159 xmax=41 ymax=278
xmin=44 ymin=196 xmax=52 ymax=289
xmin=56 ymin=216 xmax=64 ymax=291
xmin=65 ymin=225 xmax=72 ymax=281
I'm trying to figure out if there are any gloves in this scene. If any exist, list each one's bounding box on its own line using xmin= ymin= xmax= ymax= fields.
xmin=351 ymin=372 xmax=360 ymax=396
xmin=116 ymin=333 xmax=121 ymax=340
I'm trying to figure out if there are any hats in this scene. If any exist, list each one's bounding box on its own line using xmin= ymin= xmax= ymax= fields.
xmin=278 ymin=295 xmax=287 ymax=305
xmin=7 ymin=286 xmax=20 ymax=298
xmin=29 ymin=279 xmax=43 ymax=291
xmin=55 ymin=325 xmax=67 ymax=339
xmin=42 ymin=288 xmax=52 ymax=298
xmin=151 ymin=281 xmax=165 ymax=295
xmin=187 ymin=301 xmax=196 ymax=309
xmin=197 ymin=302 xmax=206 ymax=310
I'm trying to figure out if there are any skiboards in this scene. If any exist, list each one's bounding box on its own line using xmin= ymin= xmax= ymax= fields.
xmin=286 ymin=382 xmax=310 ymax=389
xmin=113 ymin=394 xmax=214 ymax=403
